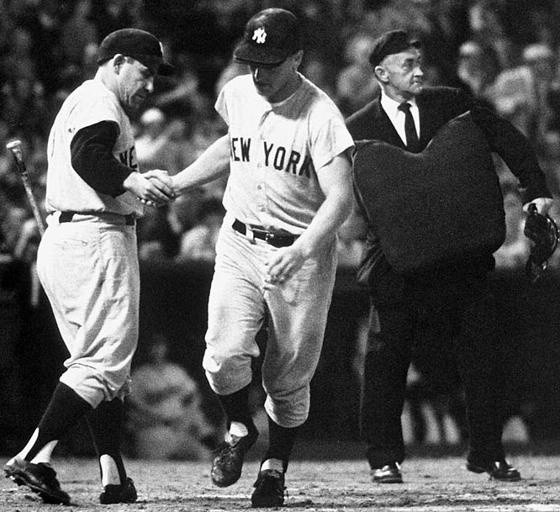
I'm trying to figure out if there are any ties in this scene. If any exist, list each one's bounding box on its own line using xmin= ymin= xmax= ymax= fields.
xmin=398 ymin=103 xmax=421 ymax=153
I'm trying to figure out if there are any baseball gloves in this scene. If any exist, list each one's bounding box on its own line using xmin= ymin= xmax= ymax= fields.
xmin=524 ymin=204 xmax=560 ymax=281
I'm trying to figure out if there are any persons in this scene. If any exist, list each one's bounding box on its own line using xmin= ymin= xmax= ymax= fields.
xmin=340 ymin=27 xmax=560 ymax=483
xmin=1 ymin=0 xmax=560 ymax=460
xmin=135 ymin=7 xmax=359 ymax=506
xmin=3 ymin=28 xmax=177 ymax=507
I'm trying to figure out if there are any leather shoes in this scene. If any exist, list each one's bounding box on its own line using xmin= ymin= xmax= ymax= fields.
xmin=369 ymin=461 xmax=403 ymax=483
xmin=467 ymin=456 xmax=521 ymax=482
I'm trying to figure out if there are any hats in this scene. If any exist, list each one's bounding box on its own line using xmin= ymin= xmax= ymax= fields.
xmin=234 ymin=8 xmax=304 ymax=64
xmin=97 ymin=27 xmax=181 ymax=77
xmin=369 ymin=30 xmax=422 ymax=65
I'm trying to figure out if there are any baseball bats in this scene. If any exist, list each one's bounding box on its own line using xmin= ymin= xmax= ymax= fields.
xmin=5 ymin=140 xmax=48 ymax=235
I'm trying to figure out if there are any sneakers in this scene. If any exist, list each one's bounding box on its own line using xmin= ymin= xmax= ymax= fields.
xmin=251 ymin=469 xmax=287 ymax=507
xmin=211 ymin=415 xmax=259 ymax=488
xmin=100 ymin=478 xmax=137 ymax=505
xmin=3 ymin=456 xmax=70 ymax=505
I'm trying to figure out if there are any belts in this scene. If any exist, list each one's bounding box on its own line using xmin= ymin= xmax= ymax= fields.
xmin=59 ymin=212 xmax=134 ymax=226
xmin=232 ymin=218 xmax=300 ymax=248
